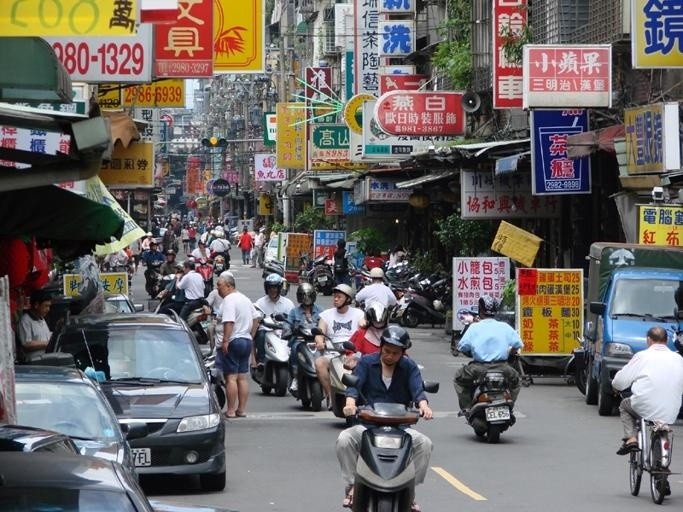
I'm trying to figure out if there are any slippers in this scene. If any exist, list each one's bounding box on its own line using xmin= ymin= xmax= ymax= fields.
xmin=616 ymin=439 xmax=639 ymax=455
xmin=343 ymin=494 xmax=354 ymax=507
xmin=411 ymin=500 xmax=420 ymax=511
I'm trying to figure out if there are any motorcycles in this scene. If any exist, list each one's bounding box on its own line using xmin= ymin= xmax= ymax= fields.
xmin=201 ymin=351 xmax=226 ymax=411
xmin=248 ymin=310 xmax=295 ymax=399
xmin=105 ymin=256 xmax=135 ymax=293
xmin=260 ymin=256 xmax=289 ymax=298
xmin=449 ymin=344 xmax=523 ymax=443
xmin=140 ymin=250 xmax=231 ymax=345
xmin=301 ymin=246 xmax=452 ymax=331
xmin=275 ymin=314 xmax=326 ymax=412
xmin=309 ymin=325 xmax=369 ymax=424
xmin=565 ymin=321 xmax=595 ymax=394
xmin=339 ymin=373 xmax=439 ymax=512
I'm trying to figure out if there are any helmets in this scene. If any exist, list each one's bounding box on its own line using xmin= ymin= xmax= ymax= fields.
xmin=479 ymin=296 xmax=498 ymax=316
xmin=365 ymin=303 xmax=388 ymax=324
xmin=264 ymin=273 xmax=283 ymax=288
xmin=166 ymin=250 xmax=176 ymax=258
xmin=296 ymin=283 xmax=317 ymax=303
xmin=381 ymin=326 xmax=411 ymax=348
xmin=370 ymin=268 xmax=385 ymax=278
xmin=216 ymin=231 xmax=222 ymax=238
xmin=332 ymin=284 xmax=353 ymax=299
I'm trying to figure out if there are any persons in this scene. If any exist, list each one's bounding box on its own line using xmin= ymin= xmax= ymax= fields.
xmin=355 ymin=268 xmax=397 ymax=321
xmin=250 ymin=272 xmax=326 ymax=391
xmin=237 ymin=228 xmax=265 ymax=268
xmin=334 ymin=239 xmax=352 ymax=285
xmin=366 ymin=246 xmax=406 ymax=270
xmin=16 ymin=288 xmax=54 ymax=362
xmin=314 ymin=284 xmax=410 ymax=410
xmin=142 ymin=217 xmax=231 ymax=292
xmin=335 ymin=326 xmax=432 ymax=510
xmin=104 ymin=245 xmax=134 ymax=280
xmin=453 ymin=295 xmax=525 ymax=417
xmin=202 ymin=271 xmax=258 ymax=417
xmin=611 ymin=326 xmax=683 ymax=495
xmin=157 ymin=260 xmax=205 ymax=323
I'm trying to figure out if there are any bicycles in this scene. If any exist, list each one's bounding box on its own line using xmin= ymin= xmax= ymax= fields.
xmin=610 ymin=383 xmax=674 ymax=505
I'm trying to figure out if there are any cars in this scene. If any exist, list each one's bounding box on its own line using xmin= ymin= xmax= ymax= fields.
xmin=0 ymin=452 xmax=153 ymax=512
xmin=13 ymin=365 xmax=139 ymax=485
xmin=0 ymin=424 xmax=238 ymax=511
xmin=45 ymin=292 xmax=144 ymax=324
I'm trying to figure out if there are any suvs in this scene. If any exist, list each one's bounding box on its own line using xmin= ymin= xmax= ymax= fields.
xmin=46 ymin=307 xmax=228 ymax=494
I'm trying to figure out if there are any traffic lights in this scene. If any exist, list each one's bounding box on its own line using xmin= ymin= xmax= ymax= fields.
xmin=201 ymin=137 xmax=228 ymax=147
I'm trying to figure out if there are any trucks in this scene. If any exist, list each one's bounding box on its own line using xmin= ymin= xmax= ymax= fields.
xmin=580 ymin=241 xmax=682 ymax=417
